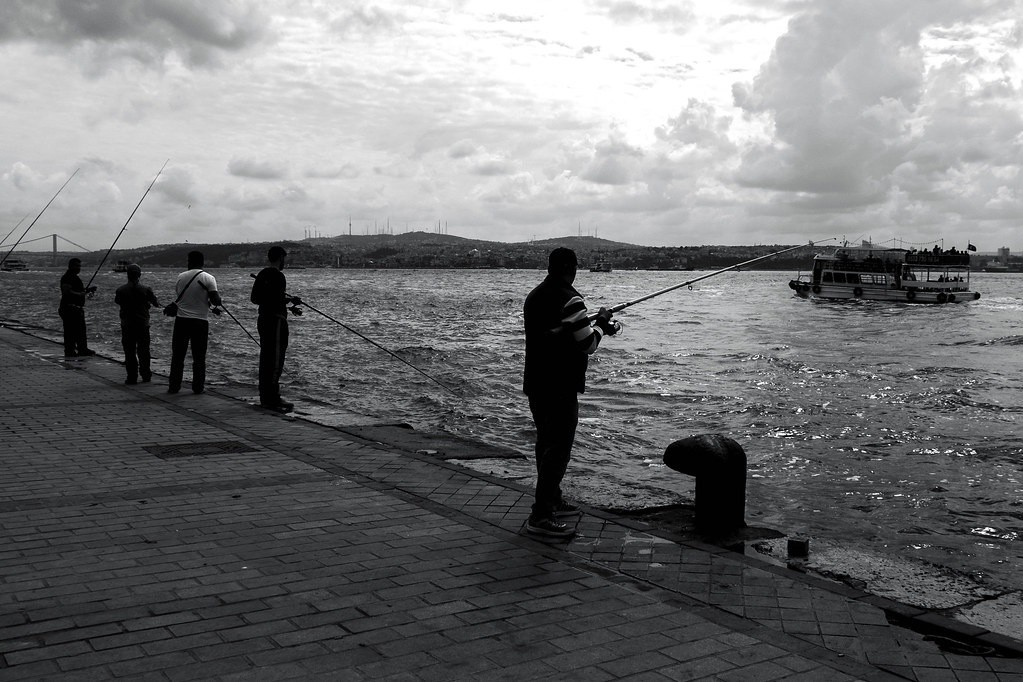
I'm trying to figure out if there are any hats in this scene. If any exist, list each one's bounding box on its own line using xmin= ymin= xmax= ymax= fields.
xmin=267 ymin=245 xmax=287 ymax=260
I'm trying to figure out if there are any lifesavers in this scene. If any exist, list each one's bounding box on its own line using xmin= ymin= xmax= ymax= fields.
xmin=803 ymin=285 xmax=980 ymax=301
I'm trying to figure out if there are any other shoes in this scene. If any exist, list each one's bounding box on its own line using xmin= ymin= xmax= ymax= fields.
xmin=260 ymin=398 xmax=294 ymax=413
xmin=125 ymin=379 xmax=137 ymax=385
xmin=167 ymin=389 xmax=179 ymax=394
xmin=142 ymin=378 xmax=152 ymax=382
xmin=193 ymin=387 xmax=206 ymax=394
xmin=78 ymin=348 xmax=96 ymax=356
xmin=65 ymin=351 xmax=86 ymax=361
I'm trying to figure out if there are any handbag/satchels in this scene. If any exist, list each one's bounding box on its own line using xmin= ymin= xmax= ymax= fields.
xmin=163 ymin=301 xmax=178 ymax=317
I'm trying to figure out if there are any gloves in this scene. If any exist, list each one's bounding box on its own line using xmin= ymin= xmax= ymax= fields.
xmin=288 ymin=304 xmax=303 ymax=316
xmin=290 ymin=295 xmax=304 ymax=306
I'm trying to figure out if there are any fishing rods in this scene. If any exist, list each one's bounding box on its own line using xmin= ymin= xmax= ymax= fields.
xmin=85 ymin=159 xmax=170 ymax=292
xmin=250 ymin=270 xmax=471 ymax=403
xmin=590 ymin=235 xmax=839 ymax=336
xmin=0 ymin=167 xmax=81 ymax=268
xmin=0 ymin=213 xmax=32 ymax=246
xmin=196 ymin=278 xmax=298 ymax=383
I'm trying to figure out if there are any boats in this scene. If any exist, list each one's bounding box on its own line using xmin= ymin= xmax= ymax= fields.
xmin=788 ymin=240 xmax=981 ymax=304
xmin=588 ymin=248 xmax=613 ymax=272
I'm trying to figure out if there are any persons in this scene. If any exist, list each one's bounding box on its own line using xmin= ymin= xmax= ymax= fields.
xmin=56 ymin=258 xmax=96 ymax=358
xmin=249 ymin=244 xmax=301 ymax=411
xmin=937 ymin=274 xmax=964 ymax=293
xmin=910 ymin=245 xmax=968 ymax=255
xmin=114 ymin=264 xmax=160 ymax=387
xmin=521 ymin=248 xmax=621 ymax=537
xmin=166 ymin=250 xmax=222 ymax=395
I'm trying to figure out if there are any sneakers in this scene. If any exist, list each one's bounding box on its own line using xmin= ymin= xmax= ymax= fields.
xmin=551 ymin=498 xmax=582 ymax=516
xmin=525 ymin=514 xmax=576 ymax=535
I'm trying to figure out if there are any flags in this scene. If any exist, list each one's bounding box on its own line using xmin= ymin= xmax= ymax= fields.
xmin=968 ymin=243 xmax=977 ymax=252
